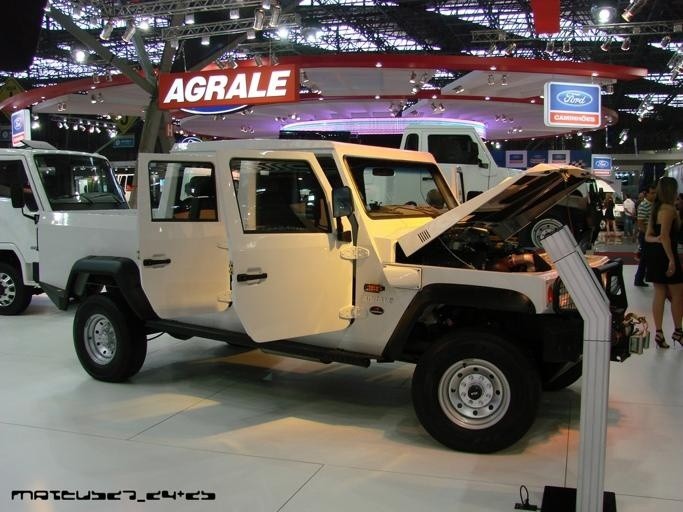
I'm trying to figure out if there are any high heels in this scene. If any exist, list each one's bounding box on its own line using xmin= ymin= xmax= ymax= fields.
xmin=672 ymin=327 xmax=683 ymax=346
xmin=655 ymin=330 xmax=669 ymax=348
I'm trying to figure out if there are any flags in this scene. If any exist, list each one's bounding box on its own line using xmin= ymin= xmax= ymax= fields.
xmin=531 ymin=0 xmax=560 ymax=33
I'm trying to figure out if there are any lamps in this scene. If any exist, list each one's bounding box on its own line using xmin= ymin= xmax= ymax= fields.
xmin=590 ymin=1 xmax=647 ymax=22
xmin=98 ymin=1 xmax=283 ymax=42
xmin=491 ymin=113 xmax=523 ymax=150
xmin=476 ymin=26 xmax=682 ymax=56
xmin=487 ymin=73 xmax=510 ymax=87
xmin=300 ymin=70 xmax=323 ymax=95
xmin=390 ymin=68 xmax=464 ymax=119
xmin=577 ymin=131 xmax=592 ymax=150
xmin=240 ymin=107 xmax=255 ymax=115
xmin=213 ymin=115 xmax=226 ymax=123
xmin=33 ymin=71 xmax=121 ymax=138
xmin=212 ymin=47 xmax=287 ymax=71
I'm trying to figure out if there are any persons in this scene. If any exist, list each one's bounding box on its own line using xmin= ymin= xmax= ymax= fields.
xmin=604 ymin=192 xmax=622 ymax=237
xmin=633 ymin=176 xmax=683 ymax=348
xmin=623 ymin=192 xmax=635 ymax=239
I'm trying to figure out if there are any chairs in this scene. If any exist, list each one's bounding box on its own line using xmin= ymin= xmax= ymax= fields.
xmin=180 ymin=176 xmax=214 ymax=219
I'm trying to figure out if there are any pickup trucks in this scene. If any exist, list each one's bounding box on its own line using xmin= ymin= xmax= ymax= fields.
xmin=39 ymin=139 xmax=627 ymax=455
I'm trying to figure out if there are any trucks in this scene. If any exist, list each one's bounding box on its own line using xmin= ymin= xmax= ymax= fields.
xmin=0 ymin=140 xmax=130 ymax=316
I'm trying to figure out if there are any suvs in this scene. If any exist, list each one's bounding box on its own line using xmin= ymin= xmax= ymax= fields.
xmin=278 ymin=118 xmax=614 ymax=253
